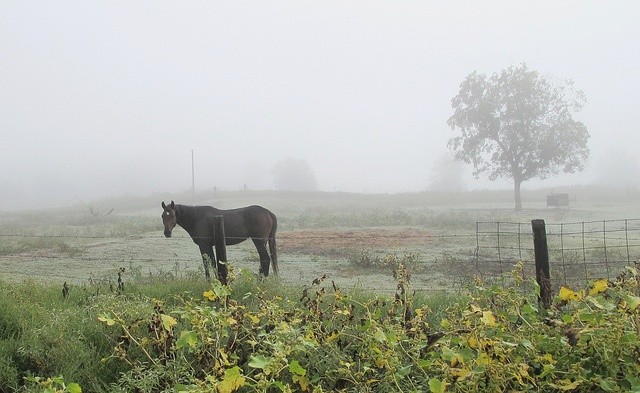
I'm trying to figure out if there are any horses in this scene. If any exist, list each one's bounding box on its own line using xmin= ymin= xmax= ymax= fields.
xmin=161 ymin=200 xmax=280 ymax=286
